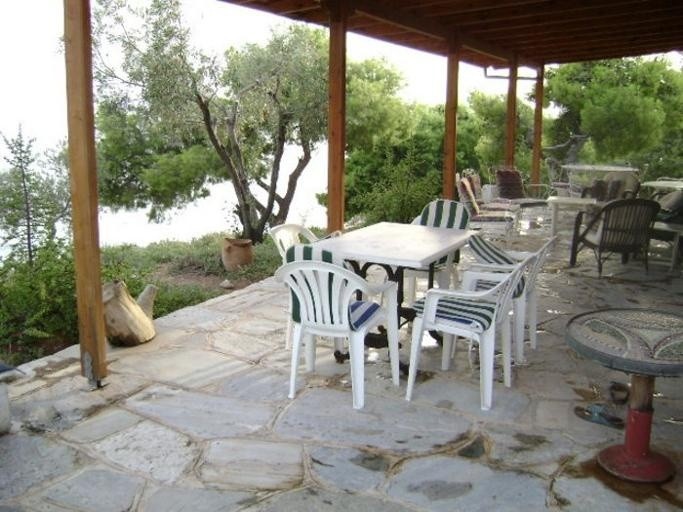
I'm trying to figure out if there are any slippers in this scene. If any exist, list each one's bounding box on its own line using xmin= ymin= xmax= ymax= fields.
xmin=575 ymin=404 xmax=624 ymax=429
xmin=611 ymin=383 xmax=628 ymax=404
